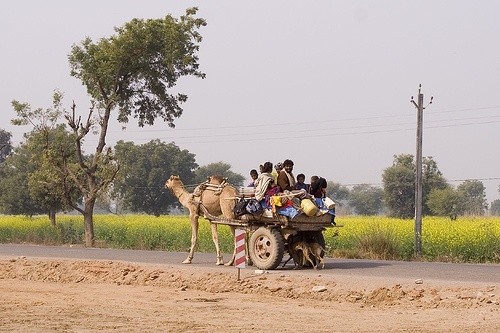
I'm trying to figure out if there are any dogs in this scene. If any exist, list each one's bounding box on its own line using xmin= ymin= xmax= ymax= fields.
xmin=283 ymin=241 xmax=331 ymax=270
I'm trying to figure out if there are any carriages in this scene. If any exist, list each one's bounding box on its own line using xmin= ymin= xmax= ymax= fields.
xmin=165 ymin=174 xmax=336 ymax=270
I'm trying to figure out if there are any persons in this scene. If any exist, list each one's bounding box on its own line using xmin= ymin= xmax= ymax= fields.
xmin=274 ymin=162 xmax=285 ymax=174
xmin=254 ymin=162 xmax=281 ymax=209
xmin=259 ymin=165 xmax=264 ymax=174
xmin=247 ymin=169 xmax=259 ymax=187
xmin=278 ymin=159 xmax=297 ymax=193
xmin=294 ymin=174 xmax=308 ymax=193
xmin=309 ymin=176 xmax=327 ymax=198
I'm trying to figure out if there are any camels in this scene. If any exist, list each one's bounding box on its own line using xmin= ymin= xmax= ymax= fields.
xmin=164 ymin=174 xmax=240 ymax=266
